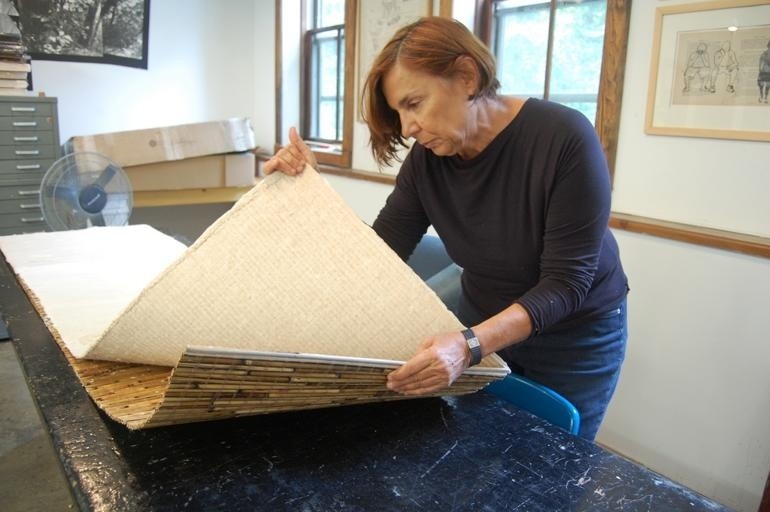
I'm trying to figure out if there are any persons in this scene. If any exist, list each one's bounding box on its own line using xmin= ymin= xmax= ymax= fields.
xmin=681 ymin=42 xmax=712 ymax=94
xmin=263 ymin=14 xmax=631 ymax=445
xmin=756 ymin=39 xmax=769 ymax=102
xmin=709 ymin=39 xmax=740 ymax=94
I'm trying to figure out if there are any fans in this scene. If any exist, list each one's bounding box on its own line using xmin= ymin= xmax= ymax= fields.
xmin=39 ymin=149 xmax=135 ymax=232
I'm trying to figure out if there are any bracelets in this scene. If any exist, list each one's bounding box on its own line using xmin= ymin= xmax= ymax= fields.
xmin=460 ymin=325 xmax=483 ymax=368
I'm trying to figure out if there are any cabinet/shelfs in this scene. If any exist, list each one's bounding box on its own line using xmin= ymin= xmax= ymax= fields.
xmin=0 ymin=96 xmax=62 ymax=235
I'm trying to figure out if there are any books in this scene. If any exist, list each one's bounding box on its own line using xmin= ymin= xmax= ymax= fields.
xmin=1 ymin=39 xmax=33 ymax=97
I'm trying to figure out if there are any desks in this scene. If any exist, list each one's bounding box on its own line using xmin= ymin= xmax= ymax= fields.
xmin=0 ymin=223 xmax=739 ymax=512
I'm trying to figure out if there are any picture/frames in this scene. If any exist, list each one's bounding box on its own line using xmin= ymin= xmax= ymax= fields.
xmin=643 ymin=1 xmax=770 ymax=142
